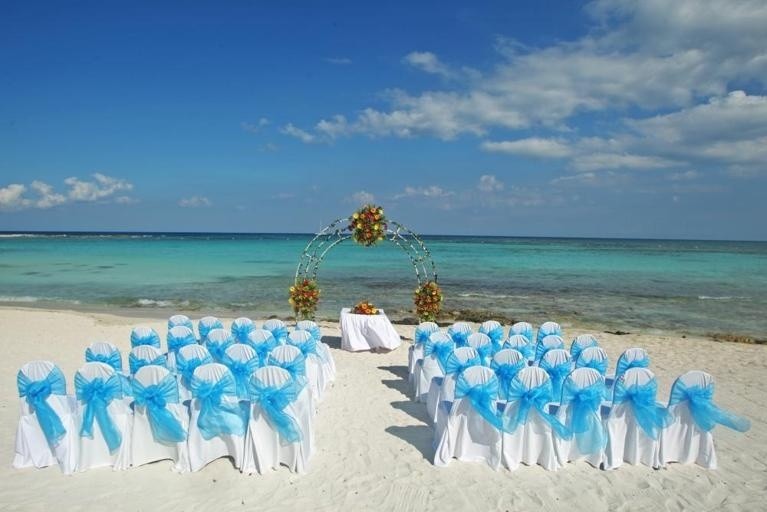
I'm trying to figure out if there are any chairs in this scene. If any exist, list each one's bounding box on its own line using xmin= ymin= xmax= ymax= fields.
xmin=498 ymin=365 xmax=573 ymax=475
xmin=242 ymin=368 xmax=308 ymax=482
xmin=655 ymin=368 xmax=751 ymax=468
xmin=73 ymin=314 xmax=335 ymax=438
xmin=602 ymin=366 xmax=677 ymax=473
xmin=15 ymin=359 xmax=74 ymax=474
xmin=411 ymin=322 xmax=653 ymax=428
xmin=130 ymin=368 xmax=187 ymax=466
xmin=186 ymin=362 xmax=241 ymax=470
xmin=70 ymin=363 xmax=133 ymax=475
xmin=429 ymin=364 xmax=522 ymax=474
xmin=552 ymin=366 xmax=611 ymax=474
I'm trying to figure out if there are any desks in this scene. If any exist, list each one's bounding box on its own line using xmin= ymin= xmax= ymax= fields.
xmin=339 ymin=308 xmax=402 ymax=351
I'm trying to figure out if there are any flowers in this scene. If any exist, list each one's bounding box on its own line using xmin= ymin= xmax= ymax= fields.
xmin=345 ymin=202 xmax=385 ymax=246
xmin=413 ymin=282 xmax=442 ymax=322
xmin=285 ymin=277 xmax=319 ymax=319
xmin=351 ymin=299 xmax=377 ymax=314
